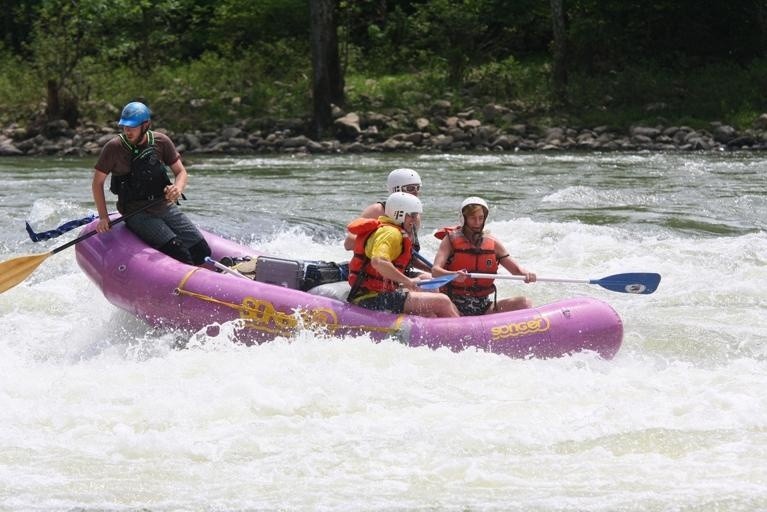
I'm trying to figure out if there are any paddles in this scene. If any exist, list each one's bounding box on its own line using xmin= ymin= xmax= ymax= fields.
xmin=0 ymin=196 xmax=166 ymax=293
xmin=464 ymin=272 xmax=661 ymax=294
xmin=397 ymin=274 xmax=459 ymax=289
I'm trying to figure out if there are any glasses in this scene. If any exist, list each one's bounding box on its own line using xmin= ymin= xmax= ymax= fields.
xmin=233 ymin=255 xmax=251 ymax=264
xmin=395 ymin=184 xmax=422 ymax=193
xmin=408 ymin=211 xmax=418 ymax=217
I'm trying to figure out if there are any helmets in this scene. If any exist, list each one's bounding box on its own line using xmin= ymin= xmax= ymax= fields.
xmin=461 ymin=196 xmax=489 ymax=216
xmin=388 ymin=167 xmax=422 ymax=195
xmin=119 ymin=100 xmax=151 ymax=128
xmin=385 ymin=191 xmax=424 ymax=224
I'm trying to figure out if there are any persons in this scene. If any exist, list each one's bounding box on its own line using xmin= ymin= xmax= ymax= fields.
xmin=344 ymin=167 xmax=440 ymax=293
xmin=432 ymin=197 xmax=536 ymax=317
xmin=92 ymin=101 xmax=211 ymax=267
xmin=347 ymin=192 xmax=459 ymax=319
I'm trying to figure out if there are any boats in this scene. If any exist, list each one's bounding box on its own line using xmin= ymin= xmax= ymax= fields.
xmin=72 ymin=212 xmax=624 ymax=366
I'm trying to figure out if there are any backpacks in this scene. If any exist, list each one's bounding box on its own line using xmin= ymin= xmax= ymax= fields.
xmin=118 ymin=129 xmax=171 ymax=198
